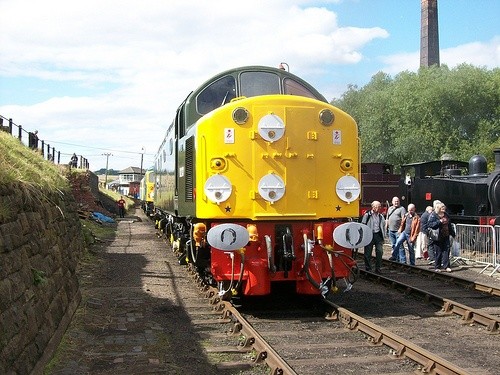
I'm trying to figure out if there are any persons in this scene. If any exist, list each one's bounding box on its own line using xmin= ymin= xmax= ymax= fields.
xmin=389 ymin=203 xmax=420 ymax=266
xmin=387 ymin=196 xmax=408 ymax=265
xmin=117 ymin=196 xmax=126 ymax=218
xmin=361 ymin=201 xmax=386 ymax=274
xmin=415 ymin=200 xmax=459 ymax=273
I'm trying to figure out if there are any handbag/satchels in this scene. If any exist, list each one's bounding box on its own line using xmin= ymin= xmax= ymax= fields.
xmin=452 ymin=239 xmax=460 ymax=257
xmin=427 ymin=228 xmax=439 ymax=241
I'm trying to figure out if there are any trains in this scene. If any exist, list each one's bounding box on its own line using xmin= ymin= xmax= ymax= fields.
xmin=361 ymin=147 xmax=500 ymax=250
xmin=128 ymin=62 xmax=374 ymax=304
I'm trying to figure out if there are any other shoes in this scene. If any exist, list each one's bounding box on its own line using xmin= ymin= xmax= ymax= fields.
xmin=389 ymin=257 xmax=396 ymax=261
xmin=446 ymin=268 xmax=451 ymax=272
xmin=366 ymin=268 xmax=372 ymax=272
xmin=434 ymin=269 xmax=441 ymax=273
xmin=375 ymin=269 xmax=383 ymax=275
